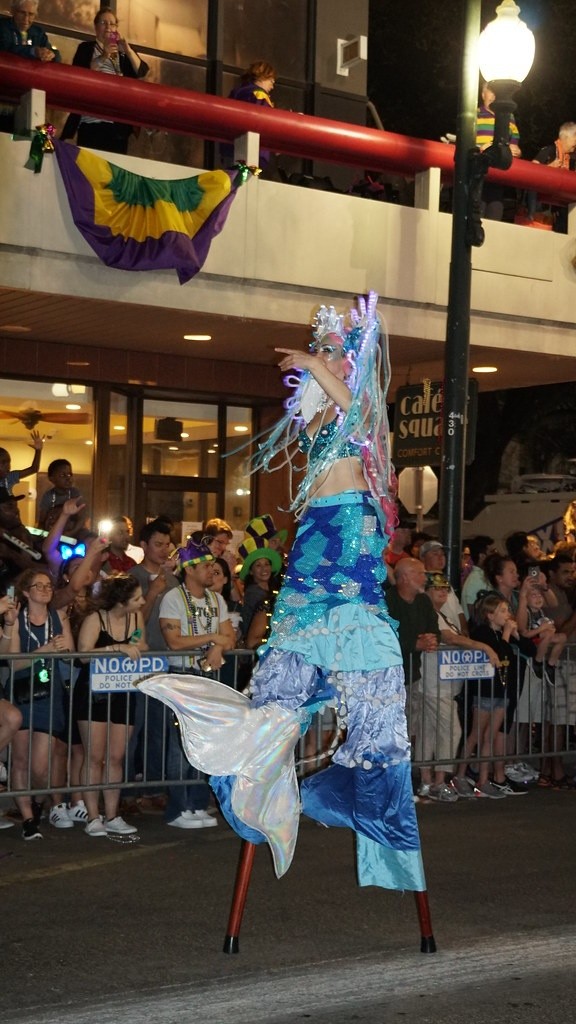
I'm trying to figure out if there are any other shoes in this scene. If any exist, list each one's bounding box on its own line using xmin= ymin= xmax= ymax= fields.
xmin=0 ymin=760 xmax=7 ymax=780
xmin=0 ymin=818 xmax=14 ymax=829
xmin=139 ymin=795 xmax=170 ymax=814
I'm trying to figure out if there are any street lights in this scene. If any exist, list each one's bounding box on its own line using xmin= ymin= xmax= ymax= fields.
xmin=438 ymin=0 xmax=536 ymax=606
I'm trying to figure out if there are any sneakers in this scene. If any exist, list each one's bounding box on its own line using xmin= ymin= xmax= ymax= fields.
xmin=415 ymin=759 xmax=541 ymax=803
xmin=544 ymin=662 xmax=556 ymax=686
xmin=527 ymin=657 xmax=544 ymax=682
xmin=32 ymin=804 xmax=47 ymax=820
xmin=85 ymin=819 xmax=107 ymax=836
xmin=538 ymin=774 xmax=554 ymax=787
xmin=21 ymin=820 xmax=43 ymax=841
xmin=104 ymin=816 xmax=137 ymax=834
xmin=67 ymin=802 xmax=103 ymax=821
xmin=166 ymin=809 xmax=217 ymax=828
xmin=49 ymin=803 xmax=74 ymax=828
xmin=552 ymin=774 xmax=576 ymax=791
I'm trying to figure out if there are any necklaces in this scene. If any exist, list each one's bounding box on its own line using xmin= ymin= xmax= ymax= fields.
xmin=96 ymin=43 xmax=118 ymax=76
xmin=22 ymin=605 xmax=54 ymax=666
xmin=181 ymin=583 xmax=213 ymax=652
xmin=492 ymin=628 xmax=507 ymax=688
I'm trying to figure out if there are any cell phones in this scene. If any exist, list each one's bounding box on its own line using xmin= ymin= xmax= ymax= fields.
xmin=6 ymin=585 xmax=15 ymax=602
xmin=529 ymin=566 xmax=539 ymax=582
xmin=199 ymin=656 xmax=226 ymax=671
xmin=110 ymin=31 xmax=118 ymax=44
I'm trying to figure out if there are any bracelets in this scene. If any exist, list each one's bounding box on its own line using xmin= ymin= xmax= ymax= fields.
xmin=4 ymin=621 xmax=15 ymax=626
xmin=540 ymin=587 xmax=550 ymax=593
xmin=2 ymin=633 xmax=11 ymax=640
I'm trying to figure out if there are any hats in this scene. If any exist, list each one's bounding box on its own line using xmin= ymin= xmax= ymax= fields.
xmin=168 ymin=538 xmax=216 ymax=567
xmin=392 ymin=518 xmax=417 ymax=530
xmin=245 ymin=514 xmax=287 ymax=544
xmin=238 ymin=537 xmax=282 ymax=580
xmin=418 ymin=541 xmax=443 ymax=559
xmin=0 ymin=487 xmax=25 ymax=503
xmin=425 ymin=574 xmax=450 ymax=588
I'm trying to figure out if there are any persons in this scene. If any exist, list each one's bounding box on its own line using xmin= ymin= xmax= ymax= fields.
xmin=218 ymin=58 xmax=305 ymax=183
xmin=136 ymin=331 xmax=411 ymax=766
xmin=526 ymin=122 xmax=576 ymax=235
xmin=448 ymin=81 xmax=521 ymax=222
xmin=0 ymin=430 xmax=287 ymax=840
xmin=496 ymin=430 xmax=533 ymax=490
xmin=382 ymin=499 xmax=576 ymax=802
xmin=59 ymin=6 xmax=151 ymax=154
xmin=0 ymin=0 xmax=62 ymax=134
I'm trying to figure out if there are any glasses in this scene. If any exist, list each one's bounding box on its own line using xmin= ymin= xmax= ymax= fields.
xmin=433 ymin=586 xmax=450 ymax=592
xmin=58 ymin=472 xmax=74 ymax=478
xmin=27 ymin=582 xmax=52 ymax=592
